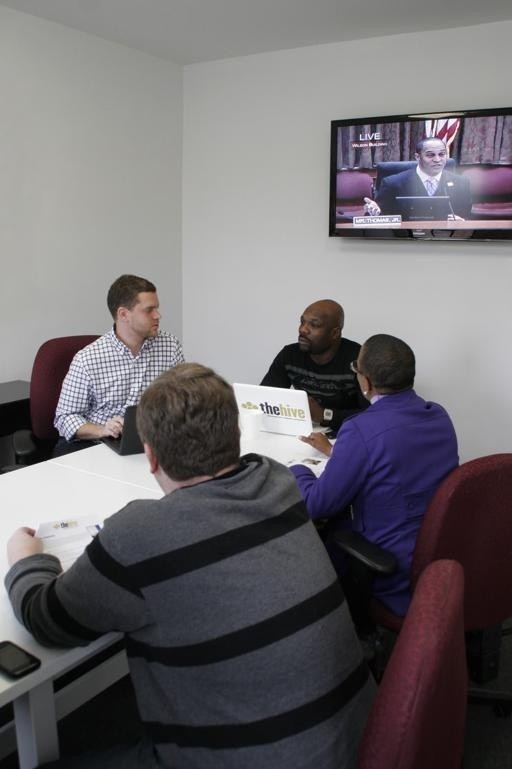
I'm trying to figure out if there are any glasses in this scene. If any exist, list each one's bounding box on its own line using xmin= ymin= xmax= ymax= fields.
xmin=350 ymin=360 xmax=367 ymax=379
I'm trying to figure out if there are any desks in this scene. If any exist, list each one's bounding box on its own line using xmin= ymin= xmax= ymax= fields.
xmin=0 ymin=429 xmax=338 ymax=769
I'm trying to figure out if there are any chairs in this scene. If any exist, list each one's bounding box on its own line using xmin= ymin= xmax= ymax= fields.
xmin=357 ymin=560 xmax=469 ymax=766
xmin=328 ymin=452 xmax=511 ymax=687
xmin=12 ymin=335 xmax=102 ymax=465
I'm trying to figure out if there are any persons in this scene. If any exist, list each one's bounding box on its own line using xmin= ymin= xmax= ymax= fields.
xmin=363 ymin=137 xmax=474 ymax=221
xmin=289 ymin=333 xmax=462 ymax=611
xmin=4 ymin=362 xmax=378 ymax=768
xmin=49 ymin=276 xmax=188 ymax=462
xmin=260 ymin=300 xmax=370 ymax=439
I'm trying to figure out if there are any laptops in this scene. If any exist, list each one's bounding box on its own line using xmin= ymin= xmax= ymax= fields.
xmin=233 ymin=381 xmax=333 ymax=439
xmin=395 ymin=195 xmax=449 ymax=223
xmin=101 ymin=406 xmax=144 ymax=455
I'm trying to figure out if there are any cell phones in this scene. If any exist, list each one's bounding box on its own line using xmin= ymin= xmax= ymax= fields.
xmin=1 ymin=640 xmax=41 ymax=681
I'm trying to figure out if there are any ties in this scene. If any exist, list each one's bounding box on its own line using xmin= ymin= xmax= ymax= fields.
xmin=427 ymin=178 xmax=438 ymax=197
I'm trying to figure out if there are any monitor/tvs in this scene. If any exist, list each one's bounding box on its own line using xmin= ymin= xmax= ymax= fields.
xmin=328 ymin=106 xmax=512 ymax=243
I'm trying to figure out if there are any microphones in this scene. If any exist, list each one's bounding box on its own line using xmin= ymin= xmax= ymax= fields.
xmin=441 ymin=180 xmax=458 ymax=220
